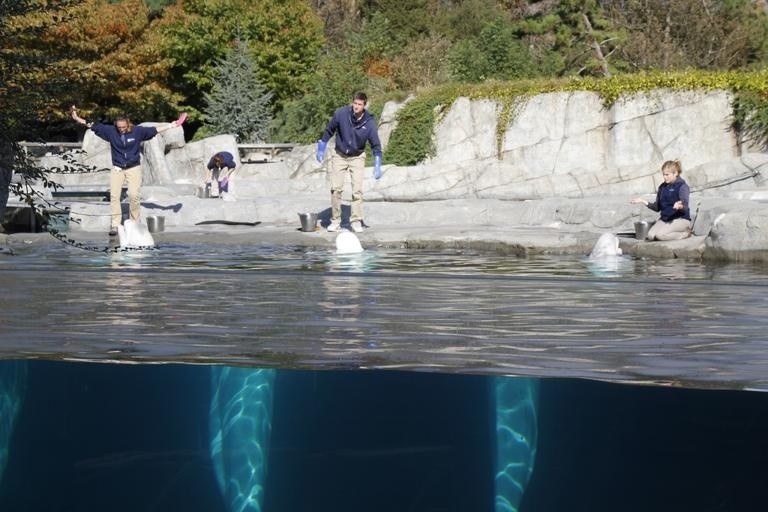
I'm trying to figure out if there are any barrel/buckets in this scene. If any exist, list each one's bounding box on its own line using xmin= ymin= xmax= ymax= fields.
xmin=298 ymin=213 xmax=318 ymax=232
xmin=146 ymin=216 xmax=165 ymax=233
xmin=198 ymin=187 xmax=209 ymax=199
xmin=634 ymin=222 xmax=653 ymax=238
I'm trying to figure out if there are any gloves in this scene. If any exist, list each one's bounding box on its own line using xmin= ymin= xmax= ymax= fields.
xmin=373 ymin=155 xmax=381 ymax=180
xmin=317 ymin=140 xmax=326 ymax=162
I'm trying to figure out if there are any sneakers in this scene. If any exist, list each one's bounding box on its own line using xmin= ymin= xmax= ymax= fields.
xmin=109 ymin=230 xmax=117 ymax=235
xmin=327 ymin=224 xmax=340 ymax=232
xmin=350 ymin=223 xmax=364 ymax=233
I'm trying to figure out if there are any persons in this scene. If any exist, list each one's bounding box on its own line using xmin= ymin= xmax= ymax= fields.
xmin=71 ymin=105 xmax=189 ymax=236
xmin=632 ymin=159 xmax=692 ymax=241
xmin=316 ymin=92 xmax=382 ymax=233
xmin=204 ymin=154 xmax=236 ymax=198
